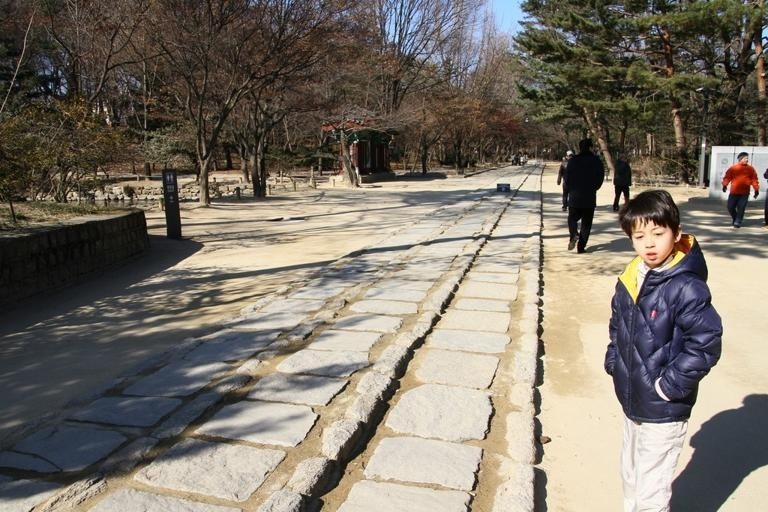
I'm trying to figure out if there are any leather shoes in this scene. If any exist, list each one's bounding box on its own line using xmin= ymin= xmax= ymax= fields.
xmin=577 ymin=240 xmax=586 ymax=253
xmin=567 ymin=233 xmax=579 ymax=250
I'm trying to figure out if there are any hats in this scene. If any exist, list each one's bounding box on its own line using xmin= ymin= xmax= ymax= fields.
xmin=566 ymin=150 xmax=576 ymax=157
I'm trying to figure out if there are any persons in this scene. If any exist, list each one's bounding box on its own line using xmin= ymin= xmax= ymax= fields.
xmin=564 ymin=138 xmax=604 ymax=253
xmin=722 ymin=152 xmax=760 ymax=228
xmin=604 ymin=190 xmax=723 ymax=511
xmin=761 ymin=167 xmax=768 ymax=225
xmin=557 ymin=149 xmax=577 ymax=213
xmin=612 ymin=153 xmax=631 ymax=211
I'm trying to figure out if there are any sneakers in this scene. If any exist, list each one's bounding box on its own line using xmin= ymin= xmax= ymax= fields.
xmin=613 ymin=206 xmax=619 ymax=212
xmin=731 ymin=218 xmax=742 ymax=227
